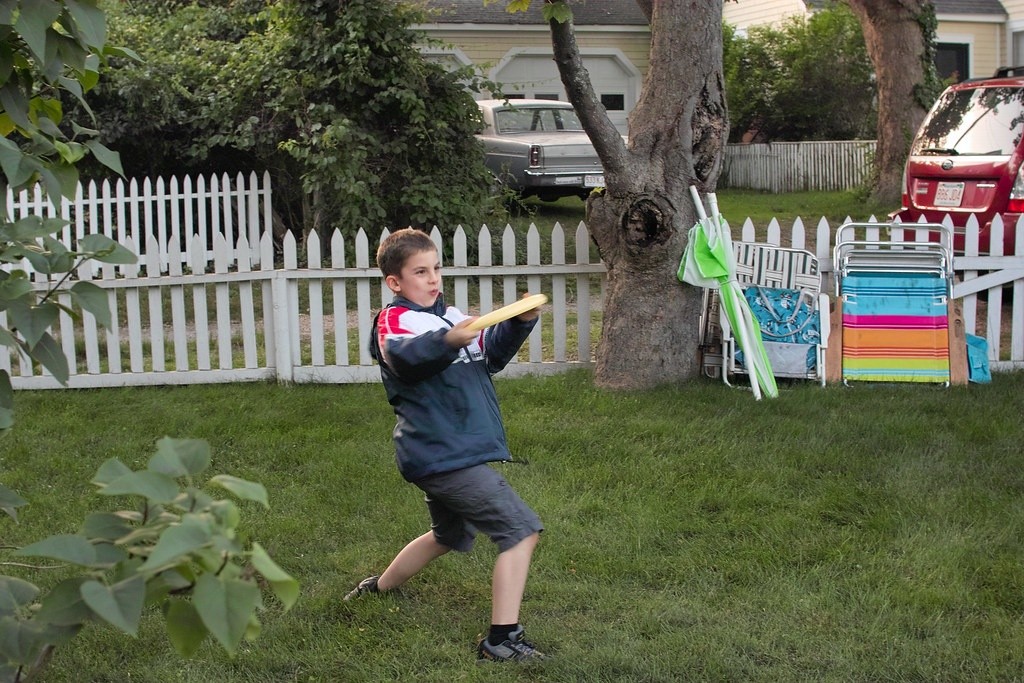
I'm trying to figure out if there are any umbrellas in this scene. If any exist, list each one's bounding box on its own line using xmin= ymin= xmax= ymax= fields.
xmin=676 ymin=185 xmax=778 ymax=398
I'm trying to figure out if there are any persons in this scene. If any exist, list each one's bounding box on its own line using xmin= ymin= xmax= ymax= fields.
xmin=342 ymin=229 xmax=550 ymax=669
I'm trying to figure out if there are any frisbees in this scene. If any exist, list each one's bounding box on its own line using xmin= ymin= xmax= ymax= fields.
xmin=464 ymin=294 xmax=549 ymax=332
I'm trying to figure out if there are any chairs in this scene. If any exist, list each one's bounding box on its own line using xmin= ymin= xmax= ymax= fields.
xmin=700 ymin=240 xmax=825 ymax=379
xmin=827 ymin=216 xmax=998 ymax=392
xmin=721 ymin=282 xmax=832 ymax=391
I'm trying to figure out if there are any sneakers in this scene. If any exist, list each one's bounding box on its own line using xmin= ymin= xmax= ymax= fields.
xmin=476 ymin=625 xmax=546 ymax=663
xmin=344 ymin=576 xmax=382 ymax=604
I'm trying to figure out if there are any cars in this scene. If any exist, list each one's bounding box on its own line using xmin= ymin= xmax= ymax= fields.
xmin=467 ymin=97 xmax=630 ymax=216
xmin=881 ymin=64 xmax=1024 ymax=306
